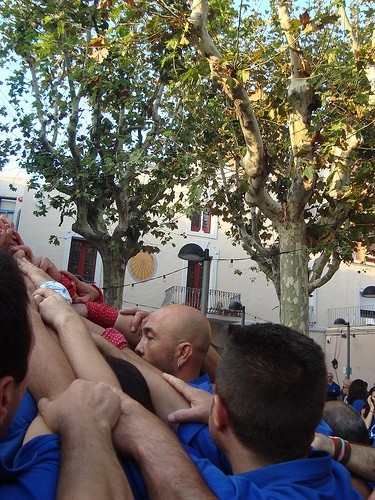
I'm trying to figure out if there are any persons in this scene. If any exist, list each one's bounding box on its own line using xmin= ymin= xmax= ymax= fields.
xmin=0 ymin=211 xmax=375 ymax=500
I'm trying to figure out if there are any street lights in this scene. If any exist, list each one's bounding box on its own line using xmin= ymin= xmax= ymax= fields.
xmin=178 ymin=242 xmax=211 ymax=318
xmin=331 ymin=317 xmax=356 ymax=381
xmin=227 ymin=301 xmax=248 ymax=327
